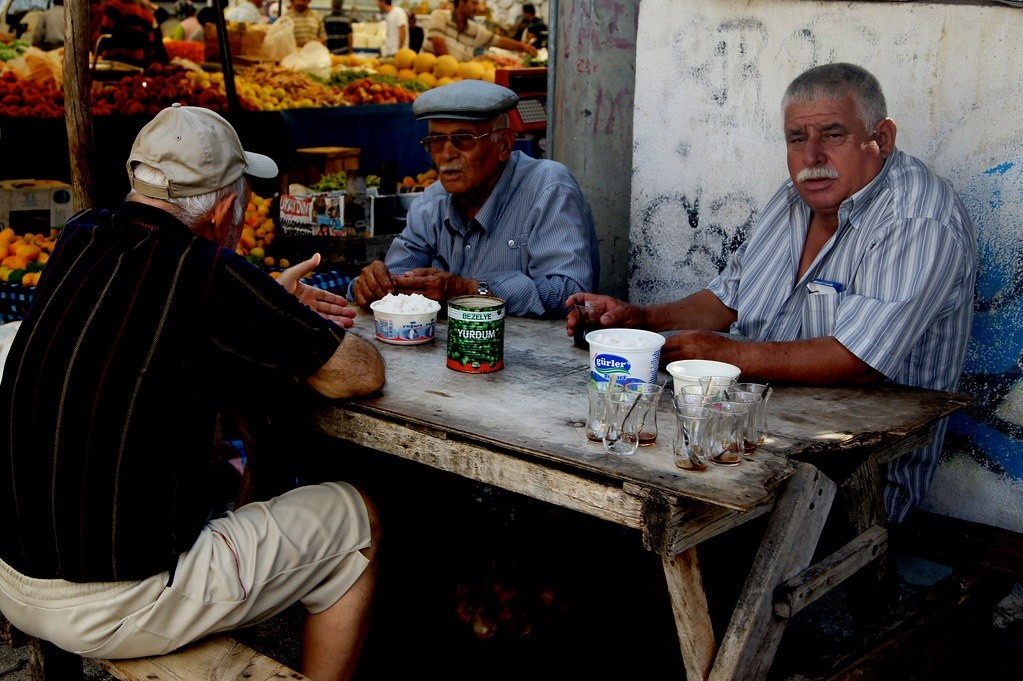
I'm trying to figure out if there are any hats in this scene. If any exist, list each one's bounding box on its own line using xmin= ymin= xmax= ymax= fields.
xmin=413 ymin=79 xmax=519 ymax=120
xmin=126 ymin=103 xmax=278 ymax=199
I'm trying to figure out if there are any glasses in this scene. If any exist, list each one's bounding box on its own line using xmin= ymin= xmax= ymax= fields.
xmin=420 ymin=130 xmax=498 ymax=153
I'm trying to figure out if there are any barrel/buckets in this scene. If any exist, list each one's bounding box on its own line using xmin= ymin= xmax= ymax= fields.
xmin=446 ymin=294 xmax=506 ymax=374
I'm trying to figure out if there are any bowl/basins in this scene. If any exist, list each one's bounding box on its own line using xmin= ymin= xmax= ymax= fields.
xmin=396 ymin=192 xmax=423 ymax=210
xmin=370 ymin=300 xmax=441 ymax=345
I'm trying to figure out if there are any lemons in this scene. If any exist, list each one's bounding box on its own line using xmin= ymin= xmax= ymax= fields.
xmin=187 ymin=72 xmax=314 ymax=110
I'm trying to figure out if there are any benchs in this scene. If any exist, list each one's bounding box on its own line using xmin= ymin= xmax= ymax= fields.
xmin=0 ymin=616 xmax=309 ymax=681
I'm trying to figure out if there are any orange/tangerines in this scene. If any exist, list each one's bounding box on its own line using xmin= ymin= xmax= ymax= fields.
xmin=0 ymin=228 xmax=60 ymax=285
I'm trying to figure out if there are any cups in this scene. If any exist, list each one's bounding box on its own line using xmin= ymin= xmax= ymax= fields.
xmin=573 ymin=300 xmax=606 ymax=351
xmin=585 ymin=328 xmax=773 ymax=473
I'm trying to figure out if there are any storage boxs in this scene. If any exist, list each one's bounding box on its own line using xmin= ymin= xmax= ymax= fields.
xmin=295 ymin=146 xmax=362 ymax=185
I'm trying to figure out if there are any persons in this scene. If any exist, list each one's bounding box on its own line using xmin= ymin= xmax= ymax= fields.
xmin=89 ymin=0 xmax=353 ymax=67
xmin=345 ymin=76 xmax=600 ymax=320
xmin=0 ymin=103 xmax=386 ymax=681
xmin=564 ymin=62 xmax=978 ymax=563
xmin=377 ymin=0 xmax=548 ymax=62
xmin=0 ymin=0 xmax=65 ymax=51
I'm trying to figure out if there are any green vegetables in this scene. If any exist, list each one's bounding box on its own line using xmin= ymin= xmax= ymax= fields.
xmin=522 ymin=54 xmax=548 ymax=67
xmin=310 ymin=170 xmax=381 ymax=192
xmin=324 ymin=68 xmax=429 ymax=93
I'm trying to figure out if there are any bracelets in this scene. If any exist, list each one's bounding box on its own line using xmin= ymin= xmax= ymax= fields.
xmin=477 ymin=281 xmax=488 ymax=295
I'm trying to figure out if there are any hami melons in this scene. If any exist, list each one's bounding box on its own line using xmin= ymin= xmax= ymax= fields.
xmin=379 ymin=47 xmax=495 ymax=87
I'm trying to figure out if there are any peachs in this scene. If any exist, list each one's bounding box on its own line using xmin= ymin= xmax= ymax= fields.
xmin=238 ymin=192 xmax=289 ymax=281
xmin=404 ymin=170 xmax=439 ymax=186
xmin=331 ymin=77 xmax=416 ymax=105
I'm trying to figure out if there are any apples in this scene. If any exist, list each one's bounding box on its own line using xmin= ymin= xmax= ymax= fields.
xmin=0 ymin=62 xmax=261 ymax=116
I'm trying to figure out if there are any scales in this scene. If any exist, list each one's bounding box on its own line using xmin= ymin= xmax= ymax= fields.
xmin=495 ymin=67 xmax=548 ymax=159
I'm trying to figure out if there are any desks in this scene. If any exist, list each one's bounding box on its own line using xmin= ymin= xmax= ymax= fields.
xmin=309 ymin=300 xmax=977 ymax=681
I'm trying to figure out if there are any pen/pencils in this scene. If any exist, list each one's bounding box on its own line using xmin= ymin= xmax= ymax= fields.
xmin=426 ymin=244 xmax=450 ymax=271
